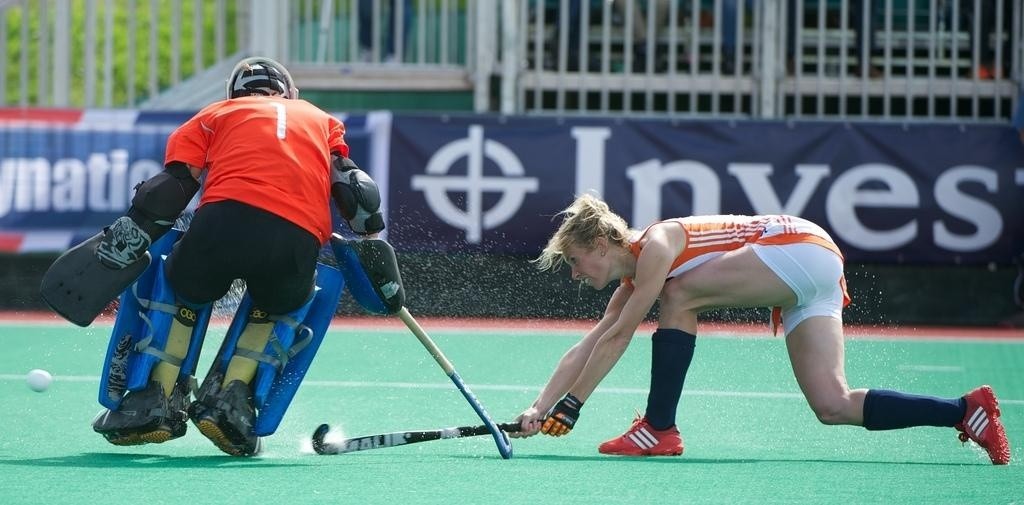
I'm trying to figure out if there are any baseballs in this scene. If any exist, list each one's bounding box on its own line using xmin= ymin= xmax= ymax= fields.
xmin=27 ymin=370 xmax=52 ymax=391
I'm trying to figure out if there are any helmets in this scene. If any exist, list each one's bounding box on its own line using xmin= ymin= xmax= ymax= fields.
xmin=225 ymin=56 xmax=299 ymax=100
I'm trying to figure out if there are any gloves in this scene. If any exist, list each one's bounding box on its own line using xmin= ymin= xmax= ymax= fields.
xmin=540 ymin=391 xmax=584 ymax=438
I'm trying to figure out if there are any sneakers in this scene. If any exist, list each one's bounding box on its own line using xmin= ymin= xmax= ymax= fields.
xmin=597 ymin=415 xmax=684 ymax=456
xmin=953 ymin=384 xmax=1010 ymax=465
xmin=89 ymin=380 xmax=190 ymax=446
xmin=197 ymin=378 xmax=261 ymax=457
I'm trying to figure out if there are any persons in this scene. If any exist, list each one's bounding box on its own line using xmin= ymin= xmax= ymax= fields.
xmin=508 ymin=193 xmax=1010 ymax=469
xmin=40 ymin=56 xmax=405 ymax=454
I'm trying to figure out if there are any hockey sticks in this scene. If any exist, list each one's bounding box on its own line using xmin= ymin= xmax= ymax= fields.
xmin=395 ymin=306 xmax=513 ymax=460
xmin=311 ymin=419 xmax=549 ymax=456
